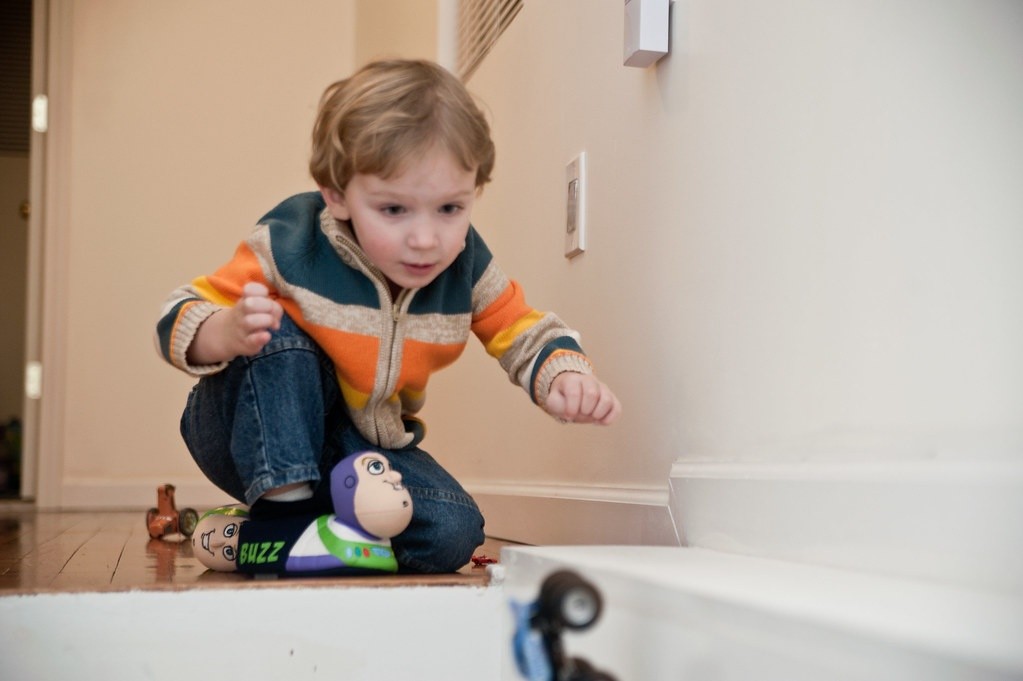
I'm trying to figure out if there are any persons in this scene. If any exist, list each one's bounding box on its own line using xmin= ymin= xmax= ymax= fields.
xmin=150 ymin=55 xmax=625 ymax=569
